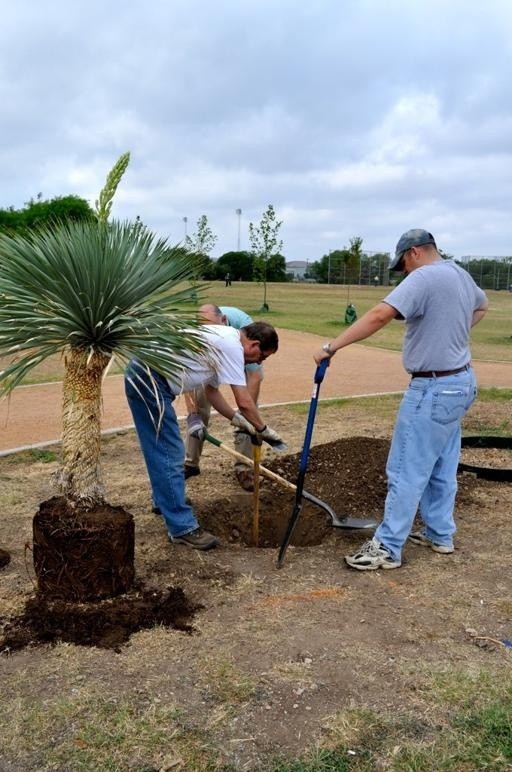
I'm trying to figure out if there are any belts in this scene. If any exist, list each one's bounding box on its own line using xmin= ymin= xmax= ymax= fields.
xmin=412 ymin=365 xmax=469 ymax=377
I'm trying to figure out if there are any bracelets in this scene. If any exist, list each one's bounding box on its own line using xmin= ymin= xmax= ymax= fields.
xmin=322 ymin=342 xmax=336 ymax=356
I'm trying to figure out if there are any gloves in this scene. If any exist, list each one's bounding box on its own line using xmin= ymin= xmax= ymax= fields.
xmin=187 ymin=412 xmax=207 ymax=441
xmin=231 ymin=412 xmax=288 ymax=451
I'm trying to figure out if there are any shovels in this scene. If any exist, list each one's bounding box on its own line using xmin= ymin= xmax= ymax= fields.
xmin=204 ymin=431 xmax=378 ymax=530
xmin=277 ymin=358 xmax=330 ymax=569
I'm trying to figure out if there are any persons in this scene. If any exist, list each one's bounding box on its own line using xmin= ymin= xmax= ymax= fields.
xmin=183 ymin=302 xmax=263 ymax=492
xmin=224 ymin=271 xmax=232 ymax=287
xmin=373 ymin=274 xmax=380 ymax=286
xmin=123 ymin=319 xmax=280 ymax=553
xmin=310 ymin=228 xmax=491 ymax=573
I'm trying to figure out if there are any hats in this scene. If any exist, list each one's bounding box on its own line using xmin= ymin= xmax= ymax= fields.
xmin=387 ymin=229 xmax=434 ymax=271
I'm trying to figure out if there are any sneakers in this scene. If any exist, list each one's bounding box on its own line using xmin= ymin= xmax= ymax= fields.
xmin=409 ymin=529 xmax=454 ymax=554
xmin=236 ymin=470 xmax=254 ymax=491
xmin=343 ymin=536 xmax=401 ymax=570
xmin=153 ymin=498 xmax=217 ymax=550
xmin=184 ymin=465 xmax=199 ymax=479
